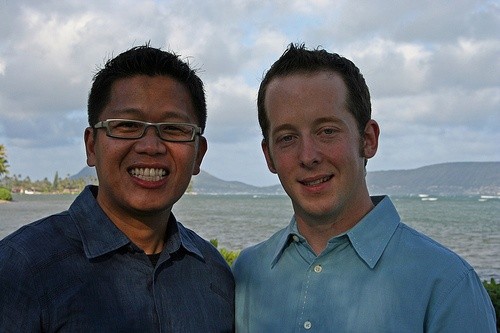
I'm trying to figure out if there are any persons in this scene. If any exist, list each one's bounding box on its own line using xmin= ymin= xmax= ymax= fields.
xmin=0 ymin=40 xmax=236 ymax=333
xmin=231 ymin=40 xmax=496 ymax=332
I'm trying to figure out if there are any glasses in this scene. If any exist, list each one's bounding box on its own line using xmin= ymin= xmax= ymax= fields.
xmin=94 ymin=118 xmax=201 ymax=142
